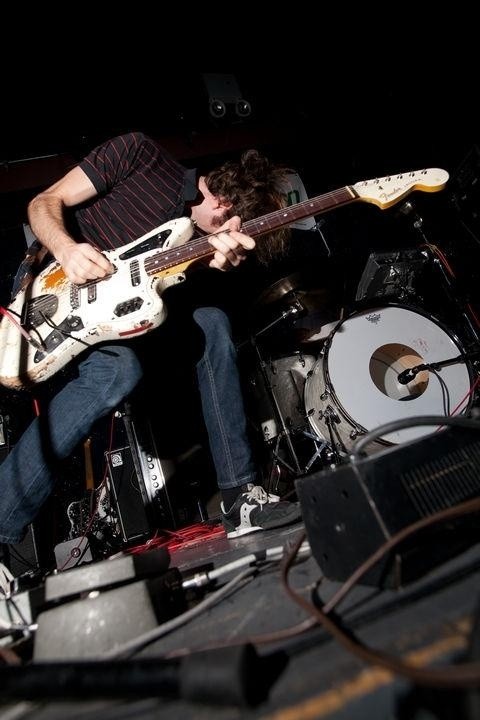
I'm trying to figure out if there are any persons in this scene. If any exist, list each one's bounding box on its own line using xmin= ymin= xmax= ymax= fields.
xmin=0 ymin=132 xmax=302 ymax=604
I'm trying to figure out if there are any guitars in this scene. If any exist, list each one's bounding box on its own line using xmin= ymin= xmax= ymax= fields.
xmin=1 ymin=166 xmax=449 ymax=392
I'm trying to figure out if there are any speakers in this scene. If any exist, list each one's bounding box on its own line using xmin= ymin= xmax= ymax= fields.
xmin=105 ymin=446 xmax=152 ymax=543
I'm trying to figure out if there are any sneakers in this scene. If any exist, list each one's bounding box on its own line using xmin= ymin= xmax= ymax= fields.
xmin=219 ymin=481 xmax=303 ymax=541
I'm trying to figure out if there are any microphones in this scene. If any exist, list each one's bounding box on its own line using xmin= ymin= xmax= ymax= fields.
xmin=399 ymin=202 xmax=424 ymax=230
xmin=397 ymin=364 xmax=426 ymax=384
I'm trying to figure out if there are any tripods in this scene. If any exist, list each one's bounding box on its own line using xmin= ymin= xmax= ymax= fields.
xmin=250 ymin=337 xmax=367 ymax=496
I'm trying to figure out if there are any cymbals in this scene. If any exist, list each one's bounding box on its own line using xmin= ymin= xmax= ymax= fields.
xmin=253 ymin=273 xmax=328 ymax=318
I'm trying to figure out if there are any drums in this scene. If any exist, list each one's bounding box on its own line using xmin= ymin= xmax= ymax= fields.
xmin=248 ymin=351 xmax=316 ymax=439
xmin=304 ymin=301 xmax=473 ymax=456
